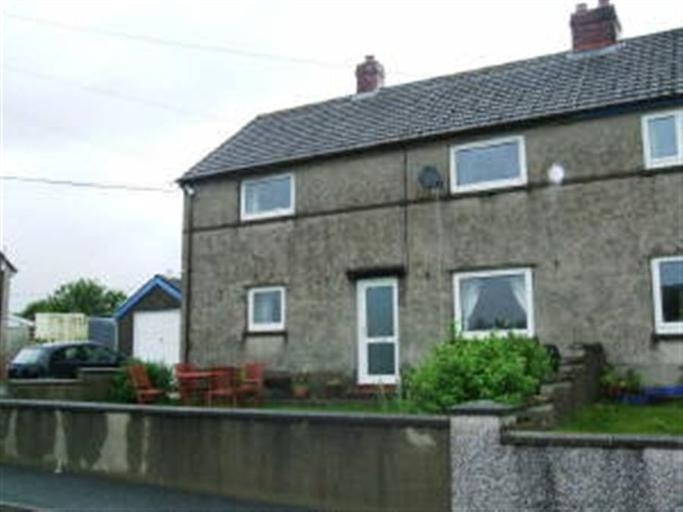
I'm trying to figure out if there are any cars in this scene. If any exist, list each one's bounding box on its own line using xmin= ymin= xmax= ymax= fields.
xmin=8 ymin=342 xmax=128 ymax=377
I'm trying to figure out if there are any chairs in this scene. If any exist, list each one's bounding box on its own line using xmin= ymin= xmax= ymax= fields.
xmin=125 ymin=358 xmax=264 ymax=408
